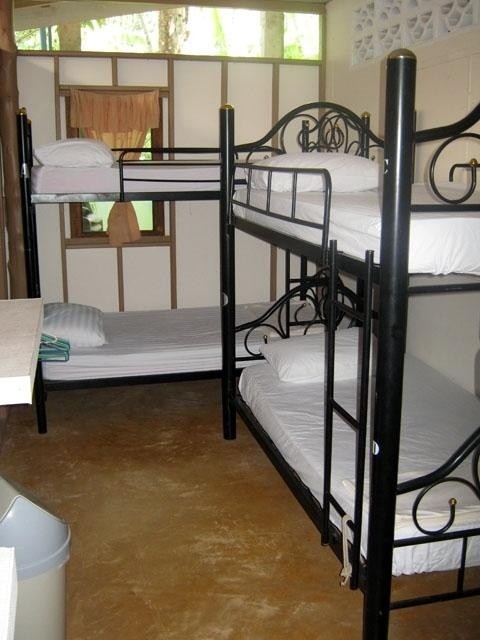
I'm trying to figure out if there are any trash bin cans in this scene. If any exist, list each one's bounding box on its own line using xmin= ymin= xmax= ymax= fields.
xmin=0 ymin=472 xmax=71 ymax=640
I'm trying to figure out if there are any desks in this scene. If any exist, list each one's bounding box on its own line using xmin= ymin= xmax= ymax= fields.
xmin=1 ymin=298 xmax=42 ymax=406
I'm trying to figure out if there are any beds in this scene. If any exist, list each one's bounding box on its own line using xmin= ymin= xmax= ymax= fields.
xmin=15 ymin=106 xmax=371 ymax=433
xmin=220 ymin=47 xmax=479 ymax=640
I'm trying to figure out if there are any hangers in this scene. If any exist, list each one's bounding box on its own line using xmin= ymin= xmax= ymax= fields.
xmin=37 ymin=331 xmax=71 ymax=362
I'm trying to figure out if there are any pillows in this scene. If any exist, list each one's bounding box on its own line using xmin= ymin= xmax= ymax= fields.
xmin=34 ymin=137 xmax=117 ymax=169
xmin=244 ymin=152 xmax=385 ymax=193
xmin=258 ymin=325 xmax=379 ymax=384
xmin=42 ymin=301 xmax=108 ymax=348
